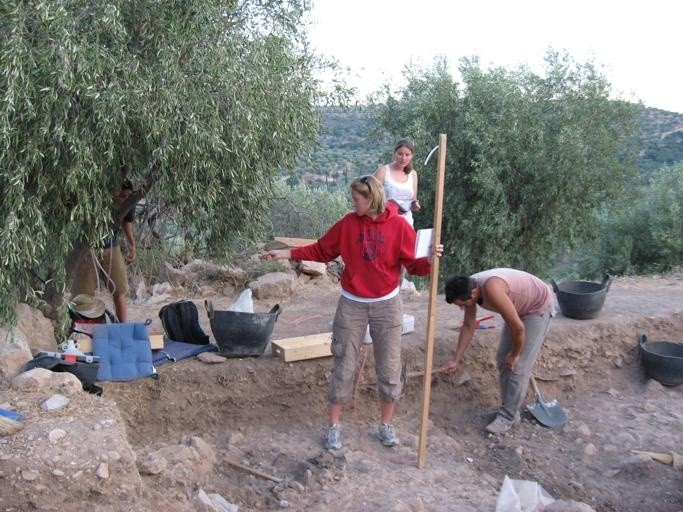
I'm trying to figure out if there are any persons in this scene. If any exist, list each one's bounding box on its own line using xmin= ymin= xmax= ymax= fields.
xmin=440 ymin=265 xmax=555 ymax=437
xmin=370 ymin=137 xmax=422 ymax=289
xmin=258 ymin=175 xmax=445 ymax=452
xmin=63 ymin=178 xmax=137 ymax=324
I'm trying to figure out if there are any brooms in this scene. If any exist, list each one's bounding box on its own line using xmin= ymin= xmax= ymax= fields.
xmin=0 ymin=414 xmax=24 ymax=437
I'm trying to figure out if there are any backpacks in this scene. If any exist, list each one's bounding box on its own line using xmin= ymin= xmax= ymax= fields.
xmin=159 ymin=299 xmax=209 ymax=345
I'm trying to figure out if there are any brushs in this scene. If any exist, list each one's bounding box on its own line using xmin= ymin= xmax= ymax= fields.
xmin=461 ymin=316 xmax=496 ymax=329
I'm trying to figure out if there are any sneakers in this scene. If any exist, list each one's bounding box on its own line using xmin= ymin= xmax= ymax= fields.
xmin=377 ymin=424 xmax=398 ymax=447
xmin=324 ymin=423 xmax=342 ymax=450
xmin=487 ymin=411 xmax=520 ymax=433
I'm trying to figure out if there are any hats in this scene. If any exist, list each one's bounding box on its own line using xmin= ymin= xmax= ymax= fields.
xmin=70 ymin=294 xmax=106 ymax=318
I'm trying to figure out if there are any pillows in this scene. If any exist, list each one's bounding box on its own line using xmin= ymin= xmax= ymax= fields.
xmin=87 ymin=320 xmax=155 ymax=384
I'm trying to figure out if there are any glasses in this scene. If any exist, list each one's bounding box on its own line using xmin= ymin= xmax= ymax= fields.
xmin=360 ymin=175 xmax=371 ymax=194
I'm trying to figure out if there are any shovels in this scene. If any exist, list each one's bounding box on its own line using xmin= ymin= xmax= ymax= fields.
xmin=527 ymin=373 xmax=566 ymax=427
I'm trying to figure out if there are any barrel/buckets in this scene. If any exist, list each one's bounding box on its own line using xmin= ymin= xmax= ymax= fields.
xmin=203 ymin=298 xmax=283 ymax=357
xmin=551 ymin=273 xmax=612 ymax=320
xmin=62 ymin=338 xmax=85 ymax=357
xmin=638 ymin=333 xmax=683 ymax=386
